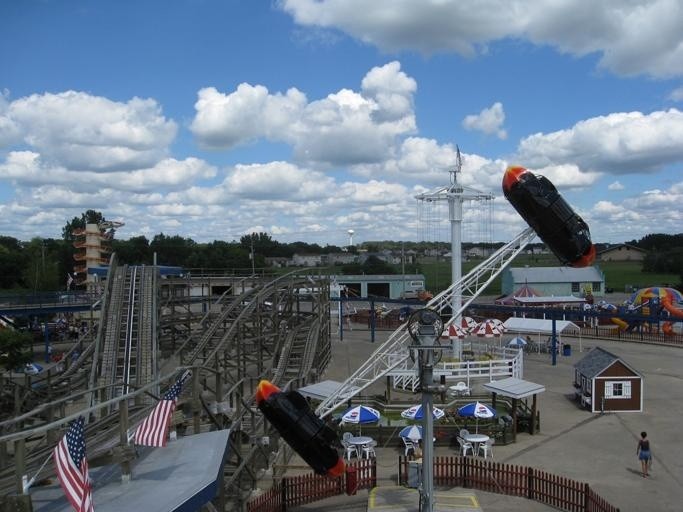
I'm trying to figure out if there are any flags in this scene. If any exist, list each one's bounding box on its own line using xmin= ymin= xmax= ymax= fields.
xmin=133 ymin=376 xmax=186 ymax=449
xmin=54 ymin=416 xmax=92 ymax=511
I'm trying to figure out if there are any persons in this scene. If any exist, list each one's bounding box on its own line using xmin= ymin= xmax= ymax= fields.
xmin=637 ymin=430 xmax=651 ymax=479
xmin=46 ymin=323 xmax=88 ymax=373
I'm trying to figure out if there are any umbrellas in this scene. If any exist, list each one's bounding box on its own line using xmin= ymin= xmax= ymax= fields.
xmin=401 ymin=405 xmax=444 ymax=421
xmin=508 ymin=336 xmax=527 ymax=346
xmin=600 ymin=303 xmax=617 ymax=311
xmin=440 ymin=315 xmax=507 ymax=361
xmin=13 ymin=363 xmax=43 ymax=375
xmin=457 ymin=401 xmax=497 ymax=455
xmin=341 ymin=405 xmax=381 ymax=436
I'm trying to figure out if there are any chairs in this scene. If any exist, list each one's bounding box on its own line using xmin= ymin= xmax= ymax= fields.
xmin=341 ymin=429 xmax=494 ymax=461
xmin=449 ymin=382 xmax=472 ymax=396
